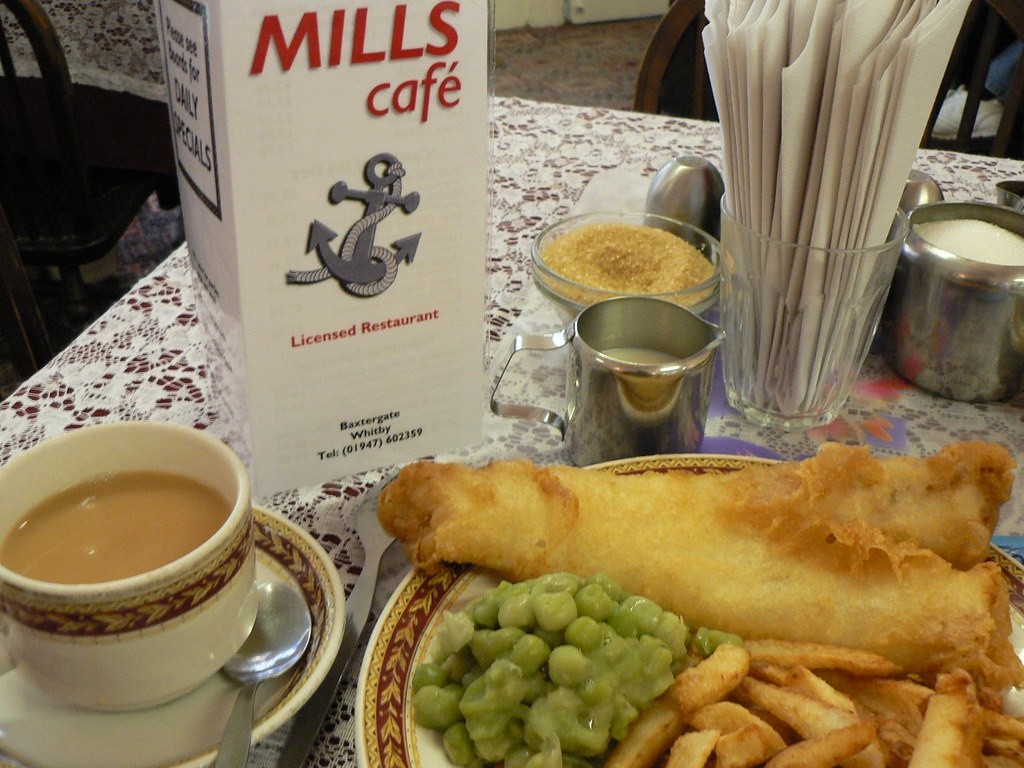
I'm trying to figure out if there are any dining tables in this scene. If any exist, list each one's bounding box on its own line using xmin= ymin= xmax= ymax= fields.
xmin=0 ymin=96 xmax=1024 ymax=768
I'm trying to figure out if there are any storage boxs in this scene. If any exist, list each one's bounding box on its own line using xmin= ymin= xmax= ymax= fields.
xmin=156 ymin=0 xmax=491 ymax=500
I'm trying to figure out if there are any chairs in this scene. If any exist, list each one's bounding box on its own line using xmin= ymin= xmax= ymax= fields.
xmin=633 ymin=0 xmax=1024 ymax=158
xmin=0 ymin=0 xmax=182 ymax=410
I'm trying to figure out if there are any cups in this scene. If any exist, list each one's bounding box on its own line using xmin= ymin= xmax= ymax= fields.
xmin=882 ymin=201 xmax=1024 ymax=403
xmin=489 ymin=294 xmax=725 ymax=469
xmin=995 ymin=180 xmax=1024 ymax=216
xmin=719 ymin=192 xmax=910 ymax=432
xmin=0 ymin=422 xmax=258 ymax=713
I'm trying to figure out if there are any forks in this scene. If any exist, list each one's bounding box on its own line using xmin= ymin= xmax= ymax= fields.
xmin=276 ymin=466 xmax=399 ymax=768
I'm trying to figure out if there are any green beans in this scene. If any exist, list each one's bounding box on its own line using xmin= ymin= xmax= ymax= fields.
xmin=409 ymin=571 xmax=745 ymax=768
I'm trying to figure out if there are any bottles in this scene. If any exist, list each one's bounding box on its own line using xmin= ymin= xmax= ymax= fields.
xmin=644 ymin=155 xmax=725 ymax=243
xmin=898 ymin=169 xmax=944 ymax=218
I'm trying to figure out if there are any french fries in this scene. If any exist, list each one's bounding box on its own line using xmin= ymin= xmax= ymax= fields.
xmin=602 ymin=638 xmax=1024 ymax=768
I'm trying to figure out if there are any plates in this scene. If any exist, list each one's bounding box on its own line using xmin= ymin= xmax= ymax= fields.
xmin=531 ymin=209 xmax=721 ymax=316
xmin=354 ymin=453 xmax=1024 ymax=768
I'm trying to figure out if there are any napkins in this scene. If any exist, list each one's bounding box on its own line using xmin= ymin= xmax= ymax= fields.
xmin=702 ymin=0 xmax=973 ymax=405
xmin=495 ymin=170 xmax=652 ymax=372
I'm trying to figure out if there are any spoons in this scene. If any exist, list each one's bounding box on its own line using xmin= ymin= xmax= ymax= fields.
xmin=0 ymin=503 xmax=346 ymax=768
xmin=215 ymin=580 xmax=313 ymax=768
